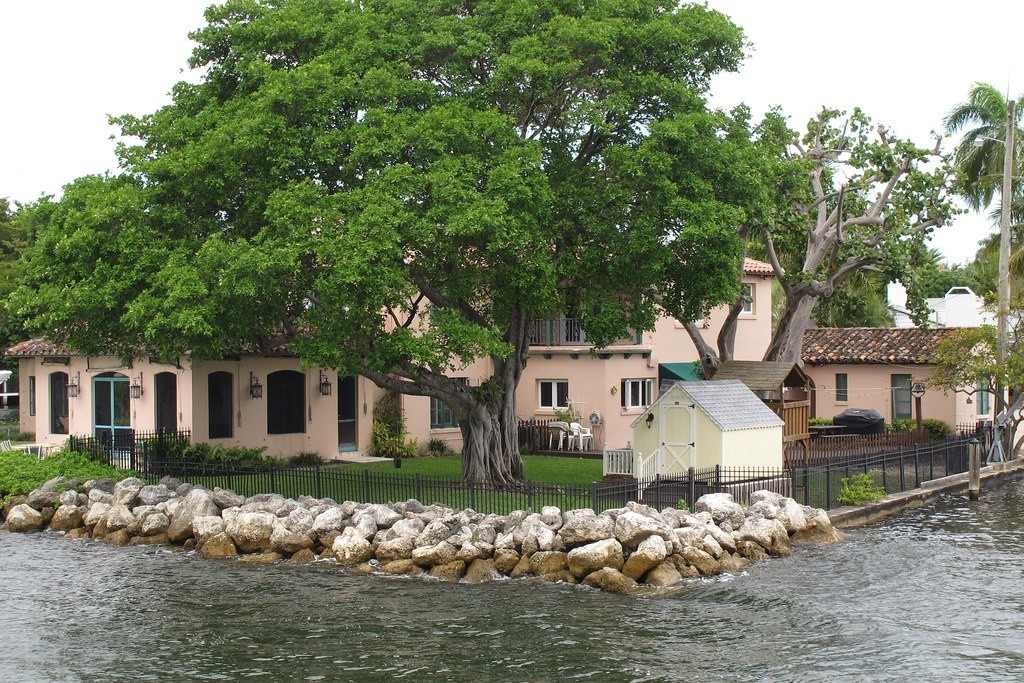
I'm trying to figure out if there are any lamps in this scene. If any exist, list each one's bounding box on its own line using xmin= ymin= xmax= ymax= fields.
xmin=320 ymin=369 xmax=333 ymax=395
xmin=131 ymin=371 xmax=143 ymax=398
xmin=248 ymin=371 xmax=265 ymax=399
xmin=66 ymin=370 xmax=81 ymax=398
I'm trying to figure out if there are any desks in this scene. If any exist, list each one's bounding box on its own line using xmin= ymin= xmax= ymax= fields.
xmin=12 ymin=443 xmax=56 ymax=459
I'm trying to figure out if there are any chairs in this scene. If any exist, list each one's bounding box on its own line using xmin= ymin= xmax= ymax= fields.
xmin=0 ymin=439 xmax=69 ymax=464
xmin=548 ymin=422 xmax=595 ymax=451
